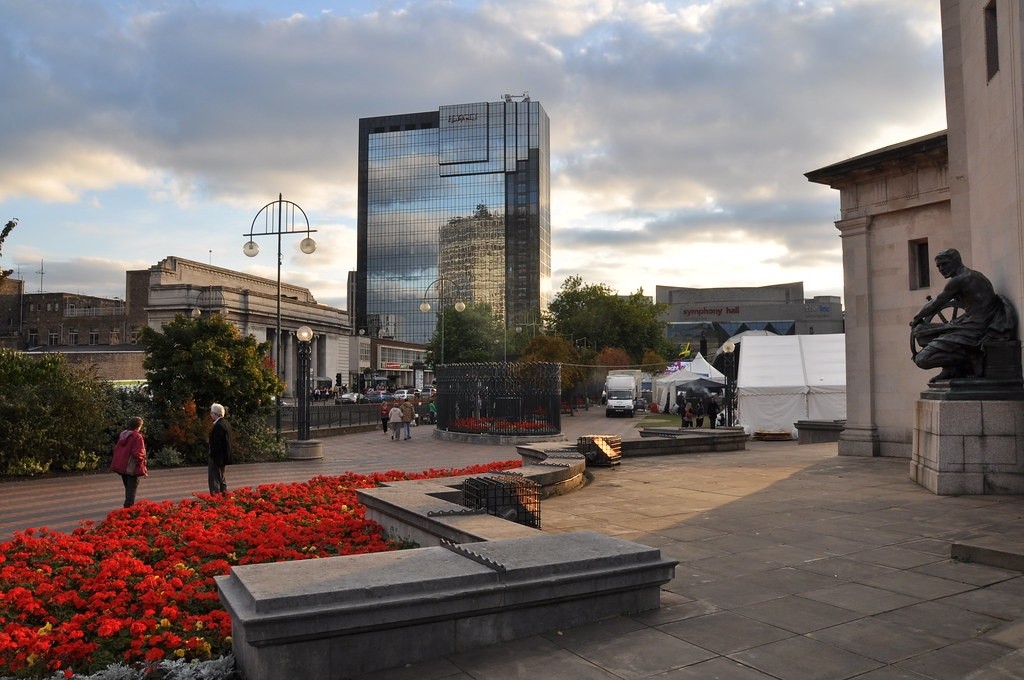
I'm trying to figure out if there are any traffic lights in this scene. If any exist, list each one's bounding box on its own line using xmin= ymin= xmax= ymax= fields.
xmin=336 ymin=373 xmax=341 ymax=386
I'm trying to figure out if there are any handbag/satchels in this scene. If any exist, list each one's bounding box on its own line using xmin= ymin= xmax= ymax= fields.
xmin=124 ymin=454 xmax=147 ymax=476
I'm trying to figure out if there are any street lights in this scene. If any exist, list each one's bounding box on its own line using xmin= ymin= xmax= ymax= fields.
xmin=418 ymin=276 xmax=466 ymax=365
xmin=242 ymin=193 xmax=318 ymax=443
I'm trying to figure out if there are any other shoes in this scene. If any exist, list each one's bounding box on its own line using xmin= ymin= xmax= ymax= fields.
xmin=404 ymin=438 xmax=407 ymax=440
xmin=391 ymin=436 xmax=394 ymax=440
xmin=697 ymin=426 xmax=701 ymax=429
xmin=408 ymin=437 xmax=411 ymax=439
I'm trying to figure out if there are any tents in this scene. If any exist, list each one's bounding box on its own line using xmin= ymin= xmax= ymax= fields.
xmin=736 ymin=330 xmax=848 ymax=436
xmin=655 ymin=352 xmax=732 ymax=416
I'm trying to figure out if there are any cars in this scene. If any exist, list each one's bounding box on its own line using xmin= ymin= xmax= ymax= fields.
xmin=369 ymin=390 xmax=394 ymax=403
xmin=394 ymin=389 xmax=417 ymax=403
xmin=334 ymin=392 xmax=368 ymax=405
xmin=418 ymin=387 xmax=437 ymax=399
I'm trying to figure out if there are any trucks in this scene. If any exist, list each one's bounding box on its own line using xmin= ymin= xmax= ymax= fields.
xmin=602 ymin=369 xmax=642 ymax=418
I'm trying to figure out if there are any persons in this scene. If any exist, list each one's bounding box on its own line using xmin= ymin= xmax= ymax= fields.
xmin=602 ymin=387 xmax=732 ymax=429
xmin=909 ymin=248 xmax=996 ymax=383
xmin=207 ymin=403 xmax=233 ymax=496
xmin=110 ymin=416 xmax=149 ymax=508
xmin=308 ymin=376 xmax=437 ymax=444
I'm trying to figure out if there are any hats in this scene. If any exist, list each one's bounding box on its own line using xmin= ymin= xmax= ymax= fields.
xmin=393 ymin=403 xmax=399 ymax=407
